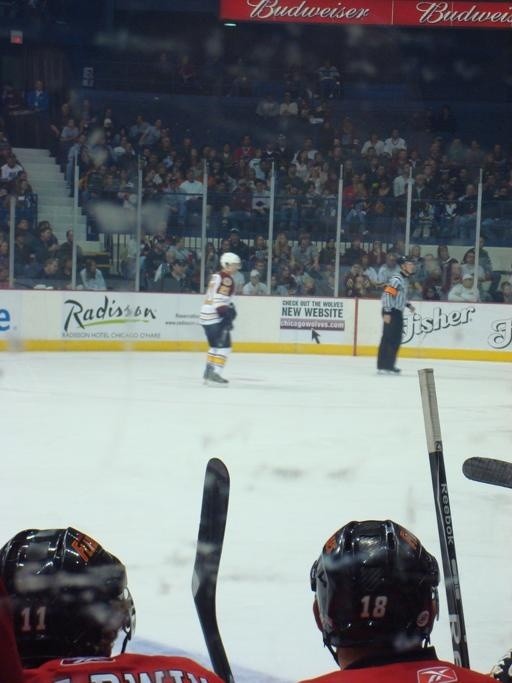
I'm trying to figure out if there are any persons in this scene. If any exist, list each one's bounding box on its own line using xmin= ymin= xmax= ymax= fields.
xmin=296 ymin=520 xmax=512 ymax=683
xmin=200 ymin=252 xmax=241 ymax=384
xmin=0 ymin=48 xmax=512 ymax=306
xmin=0 ymin=528 xmax=228 ymax=683
xmin=377 ymin=255 xmax=416 ymax=372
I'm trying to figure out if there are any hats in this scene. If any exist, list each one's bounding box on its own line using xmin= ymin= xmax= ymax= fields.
xmin=250 ymin=269 xmax=261 ymax=277
xmin=462 ymin=273 xmax=473 ymax=281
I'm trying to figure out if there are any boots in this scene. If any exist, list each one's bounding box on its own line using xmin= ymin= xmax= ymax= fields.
xmin=387 ymin=365 xmax=400 ymax=373
xmin=206 ymin=366 xmax=229 ymax=383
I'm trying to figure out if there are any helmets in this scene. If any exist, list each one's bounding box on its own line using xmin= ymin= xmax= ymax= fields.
xmin=0 ymin=527 xmax=136 ymax=669
xmin=220 ymin=252 xmax=242 ymax=272
xmin=310 ymin=520 xmax=440 ymax=649
xmin=397 ymin=255 xmax=418 ymax=275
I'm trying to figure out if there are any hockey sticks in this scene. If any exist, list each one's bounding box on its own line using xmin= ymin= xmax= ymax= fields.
xmin=462 ymin=457 xmax=512 ymax=488
xmin=191 ymin=458 xmax=235 ymax=683
